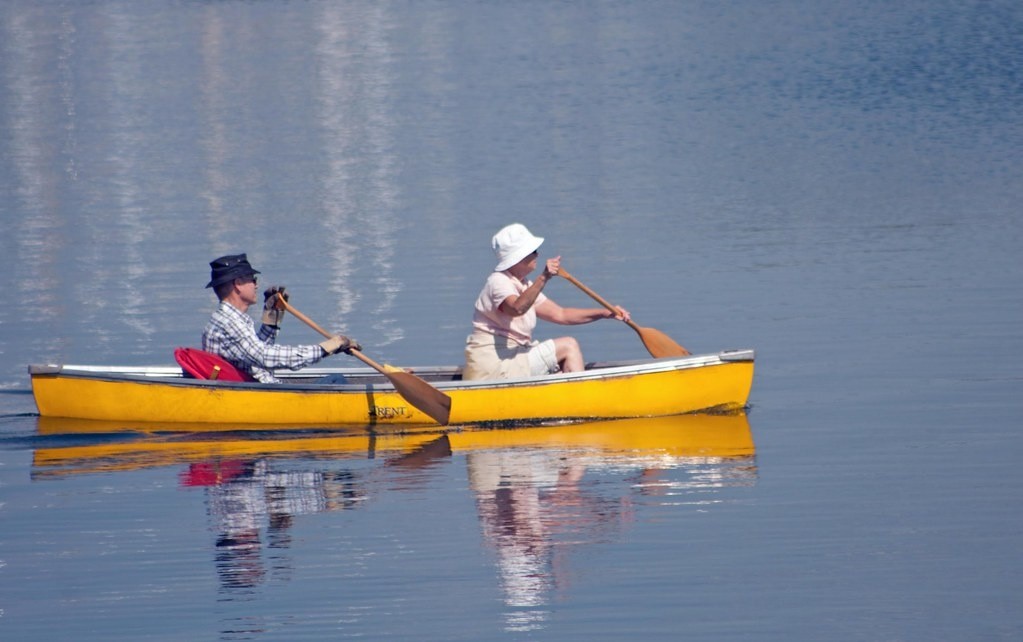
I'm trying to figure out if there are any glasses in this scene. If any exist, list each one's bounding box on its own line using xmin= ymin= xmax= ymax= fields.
xmin=241 ymin=276 xmax=257 ymax=283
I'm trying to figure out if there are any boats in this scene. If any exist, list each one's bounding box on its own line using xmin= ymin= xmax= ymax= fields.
xmin=27 ymin=347 xmax=757 ymax=429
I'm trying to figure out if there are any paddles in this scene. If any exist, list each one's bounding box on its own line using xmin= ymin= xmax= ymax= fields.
xmin=274 ymin=292 xmax=451 ymax=427
xmin=556 ymin=267 xmax=693 ymax=358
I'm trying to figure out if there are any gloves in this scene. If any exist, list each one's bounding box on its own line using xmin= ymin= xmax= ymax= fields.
xmin=317 ymin=334 xmax=363 ymax=357
xmin=260 ymin=284 xmax=288 ymax=325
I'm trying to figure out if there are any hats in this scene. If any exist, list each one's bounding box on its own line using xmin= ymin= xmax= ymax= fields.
xmin=205 ymin=253 xmax=259 ymax=289
xmin=491 ymin=224 xmax=544 ymax=273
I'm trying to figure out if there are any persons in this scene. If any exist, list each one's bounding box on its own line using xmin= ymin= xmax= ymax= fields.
xmin=200 ymin=253 xmax=362 ymax=385
xmin=460 ymin=223 xmax=631 ymax=383
